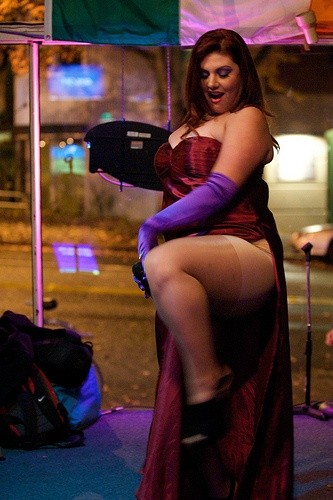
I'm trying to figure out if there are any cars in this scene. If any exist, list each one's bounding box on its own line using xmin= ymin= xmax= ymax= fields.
xmin=290 ymin=222 xmax=333 ymax=268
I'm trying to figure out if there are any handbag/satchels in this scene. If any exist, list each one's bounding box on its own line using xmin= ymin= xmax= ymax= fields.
xmin=0 ymin=314 xmax=94 ymax=452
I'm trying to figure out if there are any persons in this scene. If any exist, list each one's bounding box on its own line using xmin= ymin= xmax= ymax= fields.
xmin=132 ymin=28 xmax=295 ymax=500
